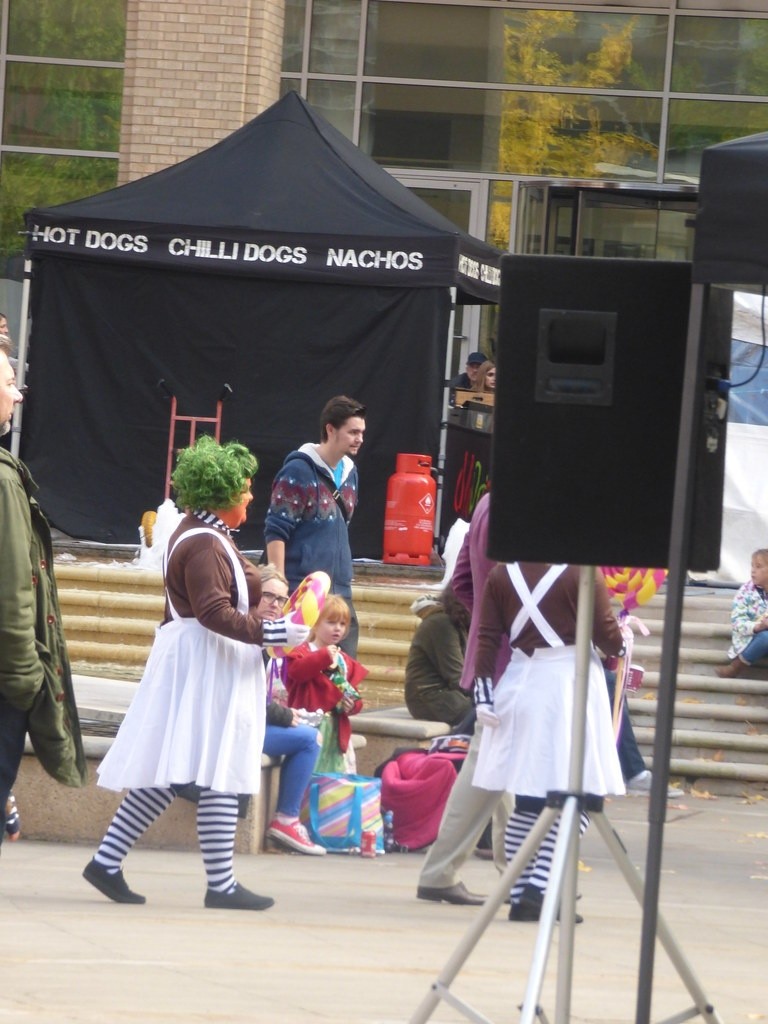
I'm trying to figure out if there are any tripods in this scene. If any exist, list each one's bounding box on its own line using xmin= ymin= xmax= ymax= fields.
xmin=406 ymin=567 xmax=719 ymax=1024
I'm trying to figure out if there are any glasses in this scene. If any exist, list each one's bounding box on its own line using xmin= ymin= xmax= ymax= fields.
xmin=262 ymin=591 xmax=288 ymax=609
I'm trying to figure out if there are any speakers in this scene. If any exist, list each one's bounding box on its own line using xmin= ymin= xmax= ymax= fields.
xmin=486 ymin=256 xmax=734 ymax=571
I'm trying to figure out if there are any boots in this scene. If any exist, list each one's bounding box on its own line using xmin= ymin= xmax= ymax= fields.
xmin=716 ymin=655 xmax=750 ymax=678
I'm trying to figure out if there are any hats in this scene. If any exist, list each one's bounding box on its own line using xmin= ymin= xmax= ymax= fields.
xmin=467 ymin=353 xmax=487 ymax=364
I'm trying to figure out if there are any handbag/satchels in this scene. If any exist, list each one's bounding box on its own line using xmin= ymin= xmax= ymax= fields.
xmin=302 ymin=771 xmax=385 ymax=855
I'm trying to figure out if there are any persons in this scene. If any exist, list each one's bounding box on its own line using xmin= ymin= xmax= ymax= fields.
xmin=254 ymin=564 xmax=327 ymax=855
xmin=280 ymin=594 xmax=370 ymax=774
xmin=603 ymin=669 xmax=684 ymax=797
xmin=0 ymin=334 xmax=86 ymax=853
xmin=81 ymin=435 xmax=311 ymax=908
xmin=416 ymin=496 xmax=510 ymax=904
xmin=404 ymin=579 xmax=474 ymax=770
xmin=715 ymin=547 xmax=768 ymax=677
xmin=459 ymin=561 xmax=626 ymax=923
xmin=454 ymin=352 xmax=496 ymax=390
xmin=258 ymin=395 xmax=366 ymax=659
xmin=0 ymin=313 xmax=8 ymax=335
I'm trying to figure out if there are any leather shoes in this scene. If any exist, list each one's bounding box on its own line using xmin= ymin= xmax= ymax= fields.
xmin=416 ymin=881 xmax=489 ymax=905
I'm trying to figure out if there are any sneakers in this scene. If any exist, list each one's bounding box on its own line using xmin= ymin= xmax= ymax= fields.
xmin=626 ymin=773 xmax=685 ymax=798
xmin=83 ymin=856 xmax=145 ymax=904
xmin=204 ymin=882 xmax=274 ymax=910
xmin=267 ymin=820 xmax=328 ymax=856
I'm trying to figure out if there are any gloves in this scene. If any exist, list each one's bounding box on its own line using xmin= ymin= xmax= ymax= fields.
xmin=475 ymin=703 xmax=501 ymax=728
xmin=284 ymin=611 xmax=311 ymax=646
xmin=596 ymin=646 xmax=615 ymax=659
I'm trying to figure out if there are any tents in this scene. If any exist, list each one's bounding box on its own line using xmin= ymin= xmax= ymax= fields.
xmin=9 ymin=91 xmax=506 ymax=559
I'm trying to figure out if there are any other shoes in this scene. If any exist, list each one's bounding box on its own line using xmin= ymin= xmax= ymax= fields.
xmin=509 ymin=888 xmax=584 ymax=923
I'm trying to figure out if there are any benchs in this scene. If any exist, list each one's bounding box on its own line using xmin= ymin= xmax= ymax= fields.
xmin=12 ymin=708 xmax=454 ymax=855
